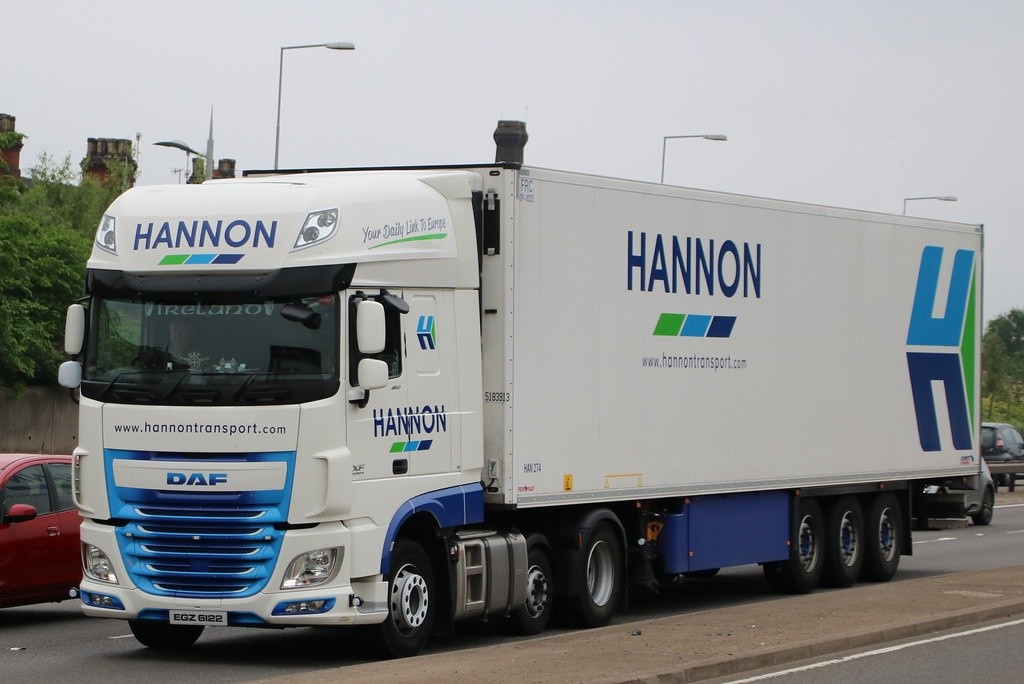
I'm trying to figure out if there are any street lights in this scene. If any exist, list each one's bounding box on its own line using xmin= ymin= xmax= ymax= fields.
xmin=657 ymin=132 xmax=730 ymax=187
xmin=271 ymin=38 xmax=358 ymax=169
xmin=900 ymin=194 xmax=957 ymax=219
xmin=153 ymin=101 xmax=222 ymax=184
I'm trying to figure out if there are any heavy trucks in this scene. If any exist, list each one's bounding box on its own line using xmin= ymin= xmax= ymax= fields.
xmin=57 ymin=117 xmax=990 ymax=658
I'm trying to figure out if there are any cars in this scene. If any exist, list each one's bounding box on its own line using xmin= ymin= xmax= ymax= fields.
xmin=0 ymin=452 xmax=90 ymax=609
xmin=908 ymin=456 xmax=997 ymax=526
xmin=977 ymin=422 xmax=1024 ymax=487
